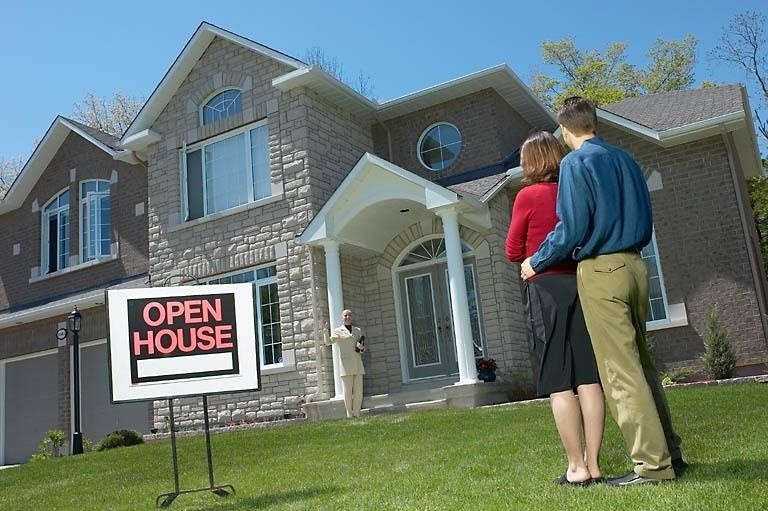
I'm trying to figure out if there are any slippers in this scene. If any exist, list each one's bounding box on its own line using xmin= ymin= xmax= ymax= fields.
xmin=552 ymin=465 xmax=592 ymax=487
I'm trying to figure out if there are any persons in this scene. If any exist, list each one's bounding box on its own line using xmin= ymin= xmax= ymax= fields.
xmin=521 ymin=96 xmax=682 ymax=486
xmin=323 ymin=310 xmax=365 ymax=418
xmin=506 ymin=131 xmax=606 ymax=486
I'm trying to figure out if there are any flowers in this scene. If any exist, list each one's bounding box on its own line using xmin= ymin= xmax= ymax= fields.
xmin=477 ymin=357 xmax=497 ymax=370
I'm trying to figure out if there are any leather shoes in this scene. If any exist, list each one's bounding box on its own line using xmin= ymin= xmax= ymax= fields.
xmin=606 ymin=469 xmax=666 ymax=485
xmin=670 ymin=456 xmax=687 ymax=479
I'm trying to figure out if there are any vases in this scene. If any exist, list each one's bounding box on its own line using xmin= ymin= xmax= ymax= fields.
xmin=478 ymin=367 xmax=496 ymax=383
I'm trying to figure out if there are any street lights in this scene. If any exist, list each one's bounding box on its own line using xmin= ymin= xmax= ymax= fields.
xmin=67 ymin=304 xmax=86 ymax=457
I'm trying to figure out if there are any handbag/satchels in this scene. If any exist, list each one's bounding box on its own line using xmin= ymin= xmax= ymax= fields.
xmin=356 ymin=335 xmax=365 ymax=353
